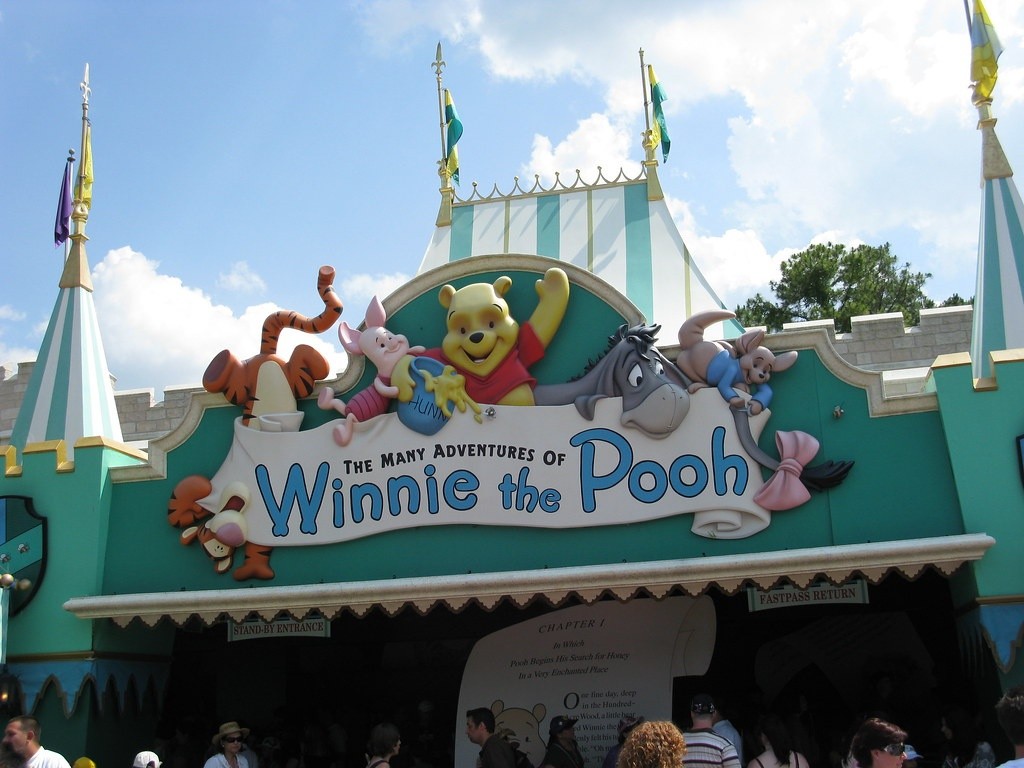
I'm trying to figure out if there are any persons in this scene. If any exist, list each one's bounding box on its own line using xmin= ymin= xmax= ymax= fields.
xmin=683 ymin=694 xmax=742 ymax=768
xmin=940 ymin=706 xmax=995 ymax=768
xmin=132 ymin=700 xmax=446 ymax=767
xmin=614 ymin=720 xmax=688 ymax=768
xmin=1 ymin=715 xmax=97 ymax=768
xmin=712 ymin=698 xmax=743 ymax=764
xmin=538 ymin=716 xmax=585 ymax=768
xmin=601 ymin=716 xmax=644 ymax=768
xmin=995 ymin=686 xmax=1024 ymax=768
xmin=745 ymin=673 xmax=924 ymax=768
xmin=466 ymin=707 xmax=516 ymax=768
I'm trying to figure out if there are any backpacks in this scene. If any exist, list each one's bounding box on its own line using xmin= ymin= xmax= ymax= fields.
xmin=503 ymin=739 xmax=536 ymax=768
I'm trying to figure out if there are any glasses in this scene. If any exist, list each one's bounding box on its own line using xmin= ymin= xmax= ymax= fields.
xmin=879 ymin=742 xmax=905 ymax=756
xmin=222 ymin=735 xmax=244 ymax=743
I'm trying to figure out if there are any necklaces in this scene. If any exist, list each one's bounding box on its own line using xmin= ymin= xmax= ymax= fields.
xmin=568 ymin=741 xmax=576 ymax=757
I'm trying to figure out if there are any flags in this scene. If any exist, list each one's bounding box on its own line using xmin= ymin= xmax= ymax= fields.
xmin=443 ymin=88 xmax=464 ymax=186
xmin=968 ymin=0 xmax=1003 ymax=104
xmin=71 ymin=122 xmax=94 ymax=208
xmin=647 ymin=64 xmax=671 ymax=164
xmin=53 ymin=162 xmax=73 ymax=249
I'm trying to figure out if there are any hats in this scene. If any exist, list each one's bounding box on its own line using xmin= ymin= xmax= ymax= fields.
xmin=617 ymin=715 xmax=644 ymax=735
xmin=690 ymin=694 xmax=716 ymax=713
xmin=212 ymin=722 xmax=250 ymax=744
xmin=73 ymin=756 xmax=96 ymax=768
xmin=904 ymin=744 xmax=924 ymax=760
xmin=548 ymin=716 xmax=579 ymax=735
xmin=132 ymin=751 xmax=162 ymax=768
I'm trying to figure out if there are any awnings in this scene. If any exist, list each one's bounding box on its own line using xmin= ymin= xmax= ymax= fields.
xmin=64 ymin=533 xmax=992 ymax=629
